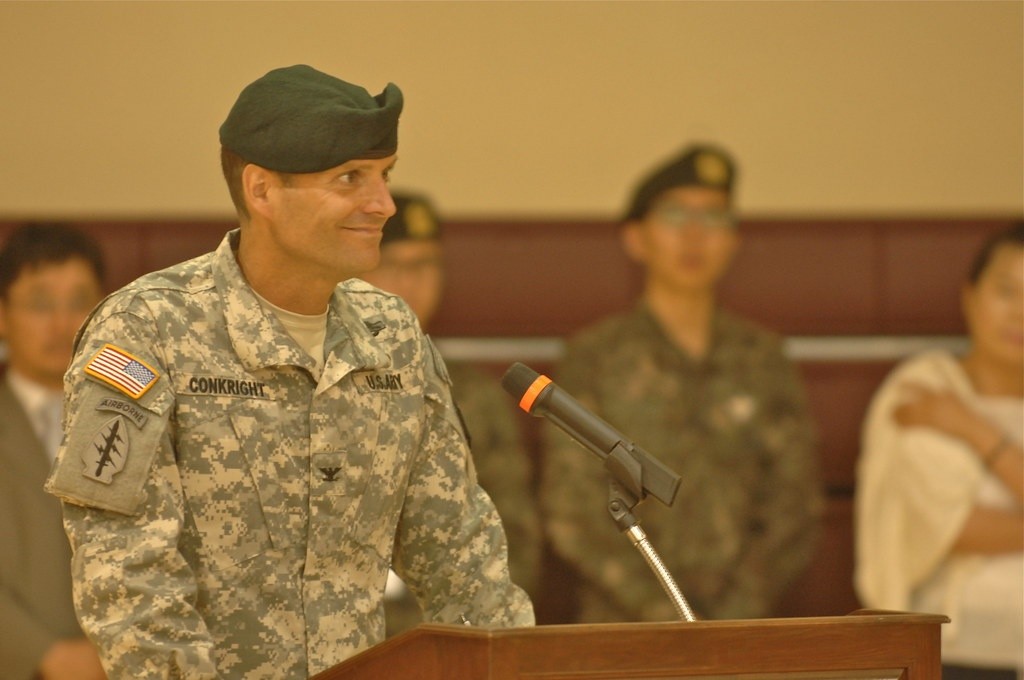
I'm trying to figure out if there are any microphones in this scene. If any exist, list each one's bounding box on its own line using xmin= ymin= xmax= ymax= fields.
xmin=501 ymin=362 xmax=683 ymax=508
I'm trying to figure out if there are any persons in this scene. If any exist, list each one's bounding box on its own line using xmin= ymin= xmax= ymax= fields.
xmin=44 ymin=63 xmax=537 ymax=680
xmin=849 ymin=224 xmax=1023 ymax=680
xmin=375 ymin=193 xmax=546 ymax=642
xmin=534 ymin=133 xmax=829 ymax=624
xmin=0 ymin=219 xmax=110 ymax=679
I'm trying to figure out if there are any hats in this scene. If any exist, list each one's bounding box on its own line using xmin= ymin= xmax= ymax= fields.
xmin=218 ymin=63 xmax=404 ymax=174
xmin=633 ymin=146 xmax=735 ymax=218
xmin=381 ymin=199 xmax=441 ymax=243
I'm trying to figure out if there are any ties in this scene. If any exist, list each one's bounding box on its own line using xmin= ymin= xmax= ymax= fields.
xmin=45 ymin=398 xmax=68 ymax=466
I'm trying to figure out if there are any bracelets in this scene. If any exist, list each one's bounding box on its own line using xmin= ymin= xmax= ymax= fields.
xmin=983 ymin=437 xmax=1013 ymax=470
